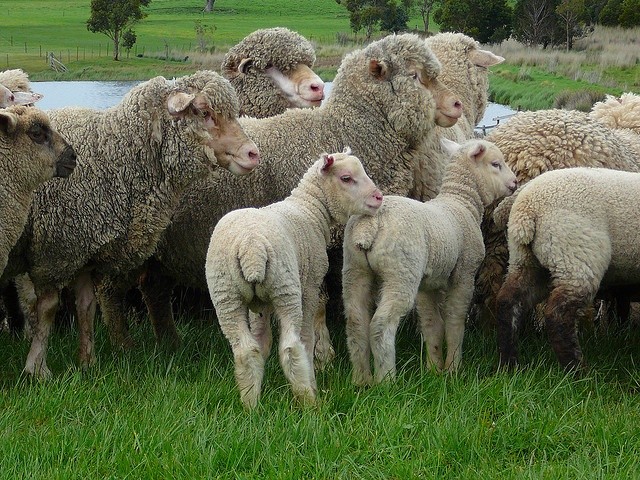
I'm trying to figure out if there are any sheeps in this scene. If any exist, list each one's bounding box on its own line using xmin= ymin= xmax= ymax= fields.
xmin=0 ymin=70 xmax=260 ymax=383
xmin=0 ymin=85 xmax=77 ymax=280
xmin=475 ymin=92 xmax=640 ymax=334
xmin=407 ymin=31 xmax=505 ymax=202
xmin=0 ymin=68 xmax=42 ymax=106
xmin=342 ymin=137 xmax=518 ymax=390
xmin=493 ymin=167 xmax=640 ymax=380
xmin=221 ymin=27 xmax=325 ymax=118
xmin=98 ymin=33 xmax=463 ymax=355
xmin=205 ymin=146 xmax=383 ymax=410
